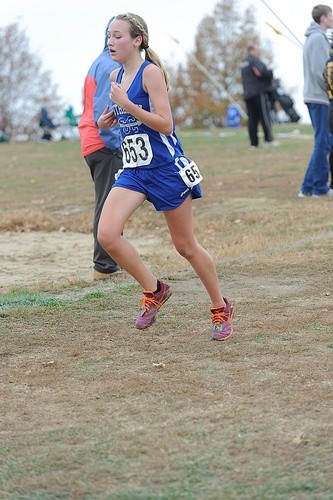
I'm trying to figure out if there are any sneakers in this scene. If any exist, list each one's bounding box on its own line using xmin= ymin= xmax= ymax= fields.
xmin=298 ymin=191 xmax=311 ymax=198
xmin=312 ymin=189 xmax=333 ymax=198
xmin=94 ymin=269 xmax=123 ymax=280
xmin=210 ymin=297 xmax=234 ymax=341
xmin=136 ymin=281 xmax=172 ymax=329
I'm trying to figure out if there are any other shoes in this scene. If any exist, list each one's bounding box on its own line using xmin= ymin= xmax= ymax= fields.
xmin=250 ymin=145 xmax=257 ymax=150
xmin=264 ymin=141 xmax=280 ymax=147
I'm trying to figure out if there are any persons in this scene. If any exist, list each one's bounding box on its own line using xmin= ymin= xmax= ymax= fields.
xmin=241 ymin=44 xmax=280 ymax=150
xmin=78 ymin=17 xmax=124 ymax=282
xmin=298 ymin=5 xmax=333 ymax=199
xmin=97 ymin=13 xmax=234 ymax=341
